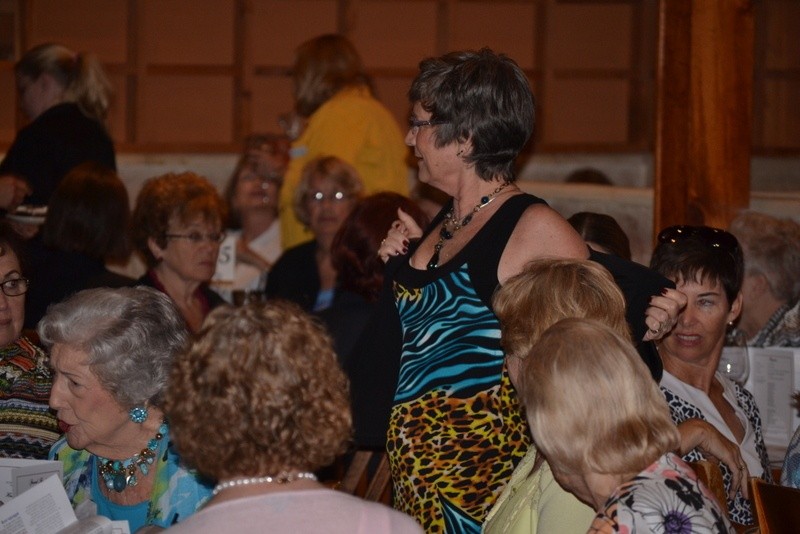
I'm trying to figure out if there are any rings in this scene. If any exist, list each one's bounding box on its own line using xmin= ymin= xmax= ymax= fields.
xmin=739 ymin=465 xmax=743 ymax=471
xmin=650 ymin=328 xmax=661 ymax=334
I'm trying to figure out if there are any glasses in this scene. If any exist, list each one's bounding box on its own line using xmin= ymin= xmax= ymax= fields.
xmin=311 ymin=189 xmax=350 ymax=202
xmin=1 ymin=276 xmax=31 ymax=296
xmin=408 ymin=116 xmax=436 ymax=128
xmin=166 ymin=232 xmax=227 ymax=244
xmin=658 ymin=227 xmax=733 ymax=254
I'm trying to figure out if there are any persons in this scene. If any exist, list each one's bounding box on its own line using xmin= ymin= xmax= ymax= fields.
xmin=0 ymin=32 xmax=799 ymax=534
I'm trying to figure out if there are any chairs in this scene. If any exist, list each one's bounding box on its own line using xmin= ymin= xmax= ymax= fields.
xmin=111 ymin=150 xmax=800 ymax=534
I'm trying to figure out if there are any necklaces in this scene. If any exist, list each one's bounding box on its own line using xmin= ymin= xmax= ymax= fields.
xmin=97 ymin=423 xmax=167 ymax=503
xmin=196 ymin=473 xmax=316 ymax=509
xmin=426 ymin=180 xmax=513 ymax=269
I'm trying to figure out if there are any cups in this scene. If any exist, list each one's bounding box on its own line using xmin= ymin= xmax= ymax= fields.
xmin=717 ymin=328 xmax=750 ymax=387
